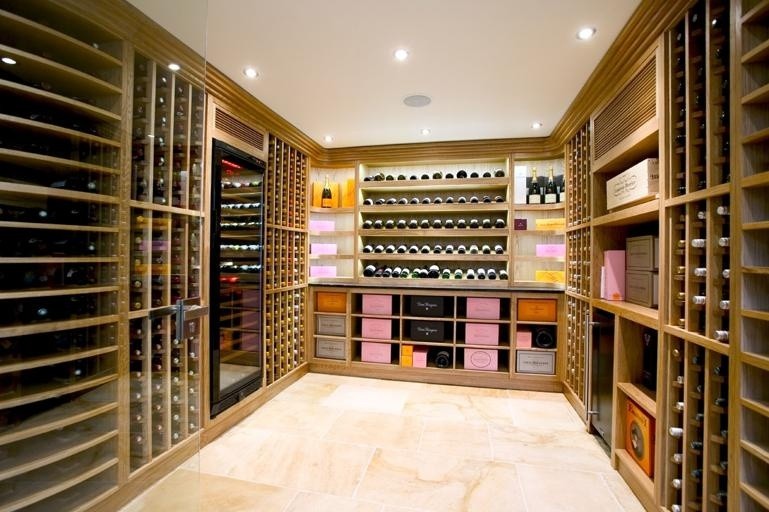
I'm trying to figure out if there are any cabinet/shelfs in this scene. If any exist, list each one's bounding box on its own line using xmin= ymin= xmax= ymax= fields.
xmin=308 ymin=145 xmax=566 ymax=392
xmin=221 ymin=185 xmax=262 ymax=368
xmin=0 ymin=0 xmax=207 ymax=512
xmin=563 ymin=1 xmax=769 ymax=511
xmin=263 ymin=128 xmax=308 ymax=405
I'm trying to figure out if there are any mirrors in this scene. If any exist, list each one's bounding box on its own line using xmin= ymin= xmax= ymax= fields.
xmin=210 ymin=136 xmax=266 ymax=419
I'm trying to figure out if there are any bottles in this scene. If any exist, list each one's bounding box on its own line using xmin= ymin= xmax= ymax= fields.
xmin=669 ymin=9 xmax=733 ymax=511
xmin=435 ymin=349 xmax=450 ymax=368
xmin=130 ymin=55 xmax=198 ymax=444
xmin=567 ymin=125 xmax=587 ymax=399
xmin=528 ymin=166 xmax=566 ymax=205
xmin=321 ymin=174 xmax=332 ymax=208
xmin=363 ymin=166 xmax=509 ymax=280
xmin=266 ymin=137 xmax=304 ymax=380
